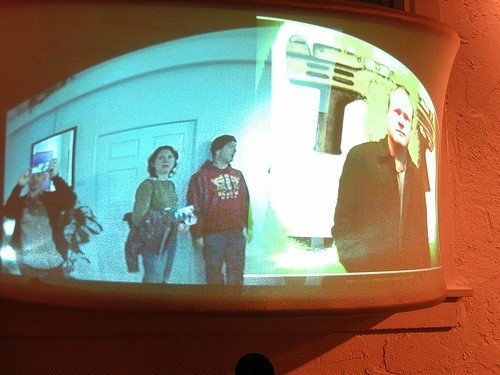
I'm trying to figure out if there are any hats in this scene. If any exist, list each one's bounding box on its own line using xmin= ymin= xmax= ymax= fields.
xmin=210 ymin=134 xmax=236 ymax=152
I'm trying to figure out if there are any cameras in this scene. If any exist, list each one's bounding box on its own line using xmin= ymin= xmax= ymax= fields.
xmin=31 ymin=161 xmax=49 ymax=174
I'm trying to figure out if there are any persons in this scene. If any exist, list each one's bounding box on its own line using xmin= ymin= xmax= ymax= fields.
xmin=186 ymin=135 xmax=251 ymax=286
xmin=330 ymin=83 xmax=434 ymax=273
xmin=129 ymin=145 xmax=194 ymax=284
xmin=234 ymin=352 xmax=275 ymax=375
xmin=3 ymin=159 xmax=78 ymax=279
